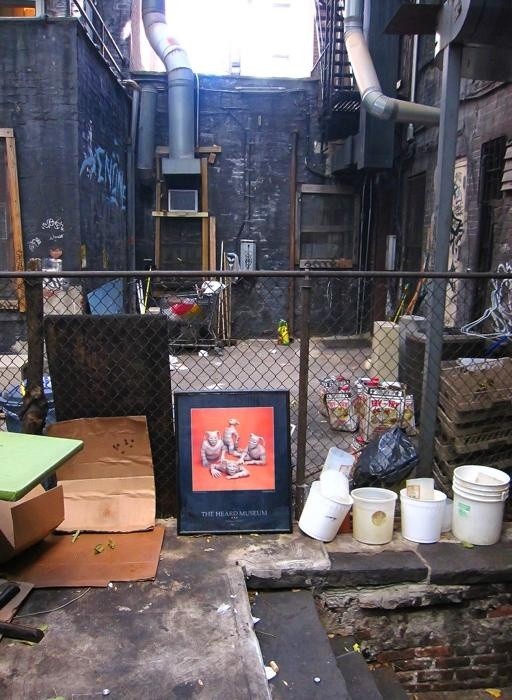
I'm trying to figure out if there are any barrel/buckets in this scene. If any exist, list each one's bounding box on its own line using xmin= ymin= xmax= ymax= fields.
xmin=350 ymin=487 xmax=398 ymax=544
xmin=398 ymin=488 xmax=447 ymax=544
xmin=295 ymin=480 xmax=354 ymax=540
xmin=450 ymin=464 xmax=511 ymax=545
xmin=0 ymin=375 xmax=58 ymax=436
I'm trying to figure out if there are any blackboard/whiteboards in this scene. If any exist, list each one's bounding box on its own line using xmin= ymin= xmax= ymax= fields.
xmin=0 ymin=131 xmax=26 ymax=307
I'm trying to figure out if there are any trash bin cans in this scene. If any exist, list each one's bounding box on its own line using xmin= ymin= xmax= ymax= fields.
xmin=405 ymin=326 xmax=484 ymax=418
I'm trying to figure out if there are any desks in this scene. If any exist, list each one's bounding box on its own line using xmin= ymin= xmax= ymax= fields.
xmin=0 ymin=430 xmax=84 ymax=643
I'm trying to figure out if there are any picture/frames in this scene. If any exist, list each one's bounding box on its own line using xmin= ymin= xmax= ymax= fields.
xmin=174 ymin=389 xmax=292 ymax=535
